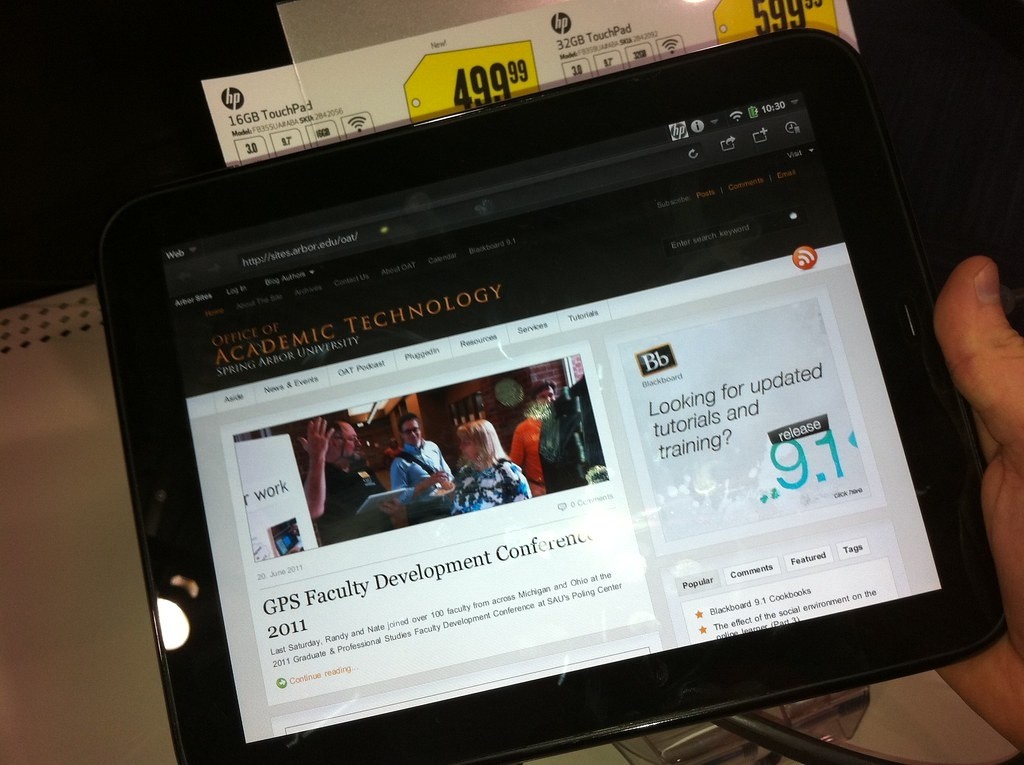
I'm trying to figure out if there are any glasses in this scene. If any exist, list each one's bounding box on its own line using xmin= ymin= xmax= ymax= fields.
xmin=402 ymin=427 xmax=420 ymax=435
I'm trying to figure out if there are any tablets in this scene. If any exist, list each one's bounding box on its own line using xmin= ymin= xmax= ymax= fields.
xmin=98 ymin=29 xmax=1007 ymax=765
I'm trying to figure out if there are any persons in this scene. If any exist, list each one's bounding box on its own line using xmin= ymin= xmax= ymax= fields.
xmin=296 ymin=380 xmax=557 ymax=546
xmin=932 ymin=256 xmax=1024 ymax=752
